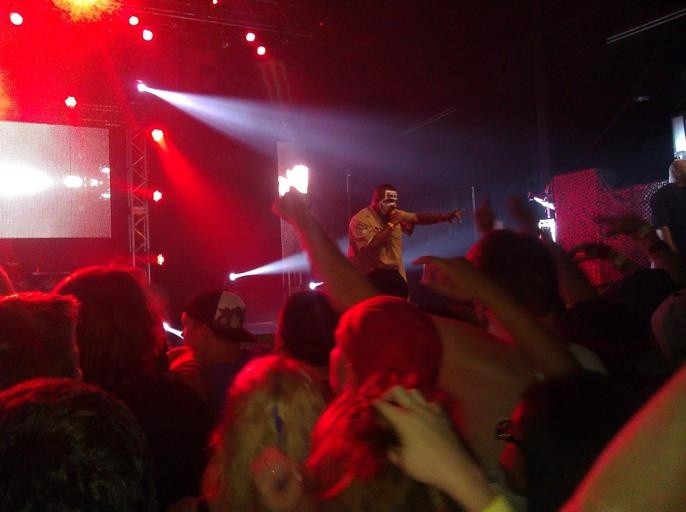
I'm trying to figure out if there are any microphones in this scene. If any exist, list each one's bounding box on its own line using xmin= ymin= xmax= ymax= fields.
xmin=391 ymin=210 xmax=412 ymax=235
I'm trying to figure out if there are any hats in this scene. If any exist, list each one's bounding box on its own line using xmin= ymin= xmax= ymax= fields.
xmin=186 ymin=289 xmax=258 ymax=343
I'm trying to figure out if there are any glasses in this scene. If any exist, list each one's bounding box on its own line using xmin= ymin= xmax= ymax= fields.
xmin=493 ymin=419 xmax=523 ymax=450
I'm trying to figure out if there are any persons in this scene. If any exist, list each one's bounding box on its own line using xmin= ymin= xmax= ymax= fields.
xmin=1 ymin=160 xmax=685 ymax=512
